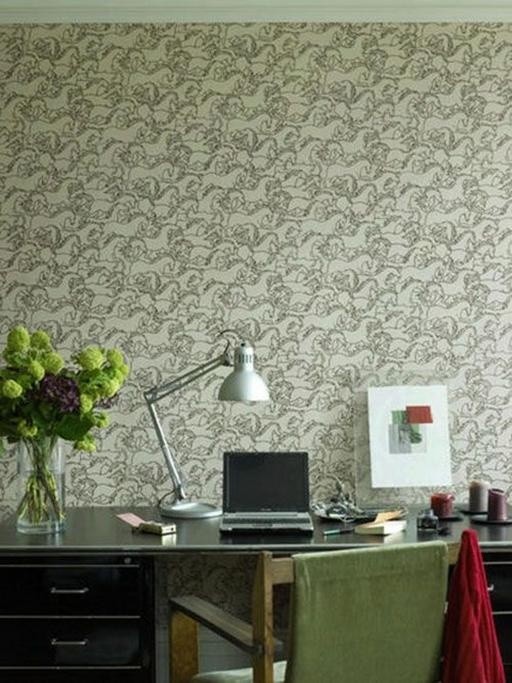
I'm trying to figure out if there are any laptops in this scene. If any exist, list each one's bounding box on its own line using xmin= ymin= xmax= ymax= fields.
xmin=219 ymin=451 xmax=314 ymax=538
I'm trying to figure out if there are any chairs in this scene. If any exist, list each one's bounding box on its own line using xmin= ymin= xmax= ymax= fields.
xmin=169 ymin=530 xmax=477 ymax=683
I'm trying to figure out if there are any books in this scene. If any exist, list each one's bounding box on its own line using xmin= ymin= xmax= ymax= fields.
xmin=355 ymin=509 xmax=407 ymax=535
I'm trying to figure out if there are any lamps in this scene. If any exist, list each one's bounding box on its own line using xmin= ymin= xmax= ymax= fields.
xmin=143 ymin=328 xmax=270 ymax=520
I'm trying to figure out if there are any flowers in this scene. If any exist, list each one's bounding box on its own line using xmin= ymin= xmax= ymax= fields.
xmin=0 ymin=326 xmax=129 ymax=527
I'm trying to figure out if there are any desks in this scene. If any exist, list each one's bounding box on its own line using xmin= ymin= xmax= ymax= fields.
xmin=0 ymin=505 xmax=512 ymax=683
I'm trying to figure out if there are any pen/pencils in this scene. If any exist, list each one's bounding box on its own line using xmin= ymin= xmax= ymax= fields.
xmin=322 ymin=528 xmax=355 ymax=535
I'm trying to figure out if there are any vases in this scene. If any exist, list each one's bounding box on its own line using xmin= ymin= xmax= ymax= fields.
xmin=17 ymin=435 xmax=65 ymax=535
